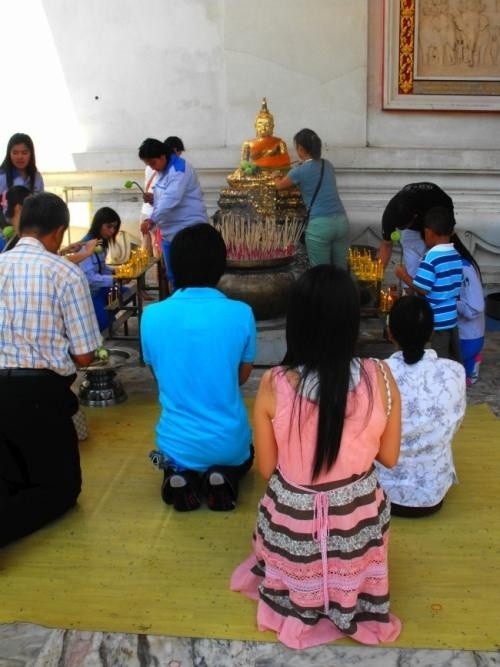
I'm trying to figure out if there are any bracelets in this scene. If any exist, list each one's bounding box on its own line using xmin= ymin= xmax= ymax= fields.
xmin=401 ymin=271 xmax=416 ymax=289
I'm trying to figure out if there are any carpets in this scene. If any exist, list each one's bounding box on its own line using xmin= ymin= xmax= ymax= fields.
xmin=1 ymin=402 xmax=499 ymax=653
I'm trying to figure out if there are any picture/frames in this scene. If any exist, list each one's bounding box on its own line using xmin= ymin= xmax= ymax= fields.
xmin=382 ymin=0 xmax=500 ymax=114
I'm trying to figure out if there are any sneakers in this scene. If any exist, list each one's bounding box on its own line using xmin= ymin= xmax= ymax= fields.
xmin=202 ymin=472 xmax=239 ymax=513
xmin=161 ymin=476 xmax=204 ymax=513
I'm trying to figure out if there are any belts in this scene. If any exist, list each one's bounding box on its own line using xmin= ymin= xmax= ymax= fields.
xmin=2 ymin=367 xmax=58 ymax=379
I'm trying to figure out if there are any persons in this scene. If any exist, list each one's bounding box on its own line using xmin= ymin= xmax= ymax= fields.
xmin=139 ymin=135 xmax=185 ymax=267
xmin=0 ymin=185 xmax=98 ymax=263
xmin=394 ymin=206 xmax=463 ymax=366
xmin=421 ymin=220 xmax=486 ymax=385
xmin=0 ymin=191 xmax=104 ymax=549
xmin=138 ymin=137 xmax=209 ymax=292
xmin=230 ymin=264 xmax=403 ymax=651
xmin=79 ymin=206 xmax=130 ymax=334
xmin=270 ymin=129 xmax=349 ymax=271
xmin=241 ymin=97 xmax=290 ymax=171
xmin=0 ymin=133 xmax=44 ymax=223
xmin=378 ymin=182 xmax=453 ymax=295
xmin=140 ymin=223 xmax=257 ymax=511
xmin=374 ymin=295 xmax=467 ymax=519
